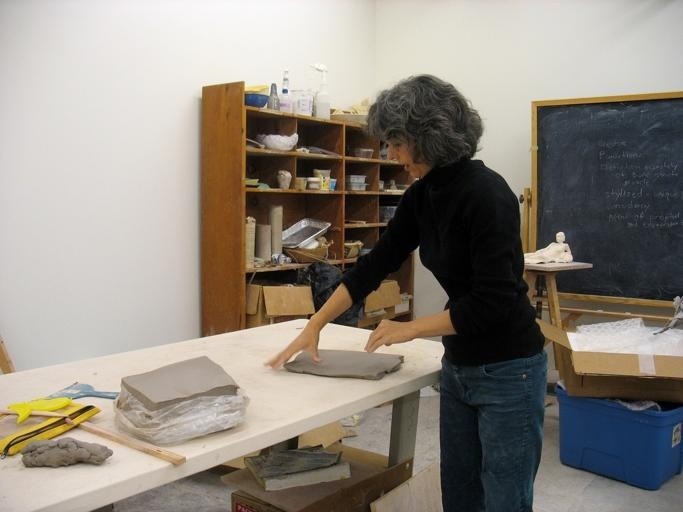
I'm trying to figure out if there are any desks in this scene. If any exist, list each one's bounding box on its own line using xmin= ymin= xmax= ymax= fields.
xmin=526 ymin=262 xmax=594 ymax=370
xmin=1 ymin=319 xmax=445 ymax=511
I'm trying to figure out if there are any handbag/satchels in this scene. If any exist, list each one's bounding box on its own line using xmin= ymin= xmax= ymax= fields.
xmin=0 ymin=397 xmax=101 ymax=459
xmin=302 ymin=262 xmax=365 ymax=328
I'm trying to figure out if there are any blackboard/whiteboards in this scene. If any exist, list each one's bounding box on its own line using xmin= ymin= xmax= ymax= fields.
xmin=530 ymin=96 xmax=683 ymax=306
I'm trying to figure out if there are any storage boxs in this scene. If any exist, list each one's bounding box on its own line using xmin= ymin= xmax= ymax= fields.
xmin=246 ymin=279 xmax=403 ymax=328
xmin=535 ymin=314 xmax=683 ymax=491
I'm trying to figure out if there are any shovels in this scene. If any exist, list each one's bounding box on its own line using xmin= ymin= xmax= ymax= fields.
xmin=43 ymin=381 xmax=119 ymax=404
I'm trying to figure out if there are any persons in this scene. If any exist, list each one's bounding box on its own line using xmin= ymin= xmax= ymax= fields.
xmin=265 ymin=73 xmax=548 ymax=512
xmin=524 ymin=231 xmax=573 ymax=264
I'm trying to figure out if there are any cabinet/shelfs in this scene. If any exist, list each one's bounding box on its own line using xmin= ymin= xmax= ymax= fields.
xmin=200 ymin=81 xmax=414 ymax=332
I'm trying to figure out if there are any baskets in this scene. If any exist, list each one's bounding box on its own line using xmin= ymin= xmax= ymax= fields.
xmin=283 ymin=246 xmax=328 ymax=263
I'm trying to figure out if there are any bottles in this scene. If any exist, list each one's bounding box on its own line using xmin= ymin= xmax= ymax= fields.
xmin=266 ymin=78 xmax=290 ymax=112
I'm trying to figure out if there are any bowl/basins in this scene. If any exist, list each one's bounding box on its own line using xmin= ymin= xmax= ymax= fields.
xmin=244 ymin=94 xmax=269 ymax=107
